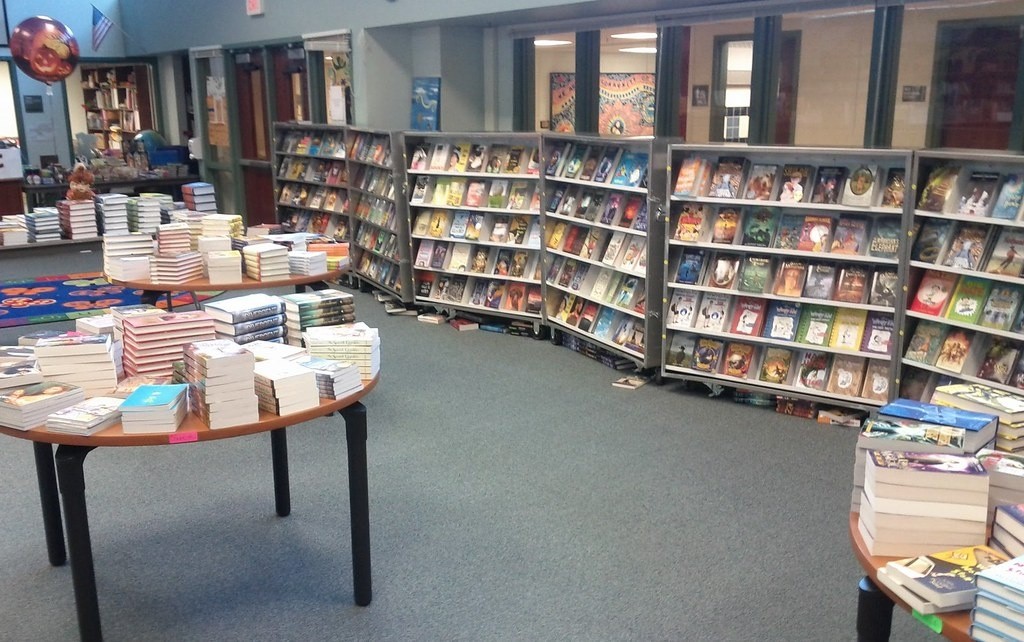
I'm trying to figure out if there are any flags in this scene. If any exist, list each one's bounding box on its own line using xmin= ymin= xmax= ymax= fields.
xmin=91 ymin=6 xmax=113 ymax=52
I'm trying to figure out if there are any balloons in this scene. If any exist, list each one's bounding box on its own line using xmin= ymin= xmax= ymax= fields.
xmin=10 ymin=16 xmax=81 ymax=87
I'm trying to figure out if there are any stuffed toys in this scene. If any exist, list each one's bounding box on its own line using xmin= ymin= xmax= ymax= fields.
xmin=67 ymin=157 xmax=95 ymax=200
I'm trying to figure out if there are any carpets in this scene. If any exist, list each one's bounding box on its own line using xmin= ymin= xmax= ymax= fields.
xmin=0 ymin=271 xmax=226 ymax=329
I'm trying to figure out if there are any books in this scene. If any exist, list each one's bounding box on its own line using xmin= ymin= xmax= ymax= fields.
xmin=844 ymin=160 xmax=1024 ymax=642
xmin=276 ymin=126 xmax=902 ymax=428
xmin=82 ymin=67 xmax=141 ymax=151
xmin=0 ymin=287 xmax=384 ymax=436
xmin=0 ymin=181 xmax=352 ymax=284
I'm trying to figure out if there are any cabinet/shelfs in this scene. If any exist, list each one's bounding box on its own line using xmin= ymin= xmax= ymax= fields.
xmin=80 ymin=62 xmax=153 ymax=158
xmin=270 ymin=120 xmax=1024 ymax=423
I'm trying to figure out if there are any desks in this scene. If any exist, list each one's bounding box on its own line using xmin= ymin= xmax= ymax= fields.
xmin=848 ymin=510 xmax=973 ymax=642
xmin=0 ymin=370 xmax=381 ymax=642
xmin=19 ymin=173 xmax=200 ymax=215
xmin=103 ymin=265 xmax=350 ymax=312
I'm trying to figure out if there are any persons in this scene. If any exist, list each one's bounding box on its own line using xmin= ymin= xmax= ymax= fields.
xmin=129 ymin=129 xmax=168 ymax=158
xmin=925 ymin=285 xmax=941 ymax=305
xmin=704 ymin=300 xmax=714 ymax=327
xmin=555 ymin=298 xmax=568 ymax=322
xmin=791 ymin=226 xmax=800 ymax=243
xmin=780 ymin=171 xmax=803 ymax=202
xmin=441 ymin=279 xmax=455 ymax=301
xmin=486 ymin=155 xmax=501 ymax=173
xmin=1001 ymin=245 xmax=1016 ymax=269
xmin=801 ymin=221 xmax=812 ymax=243
xmin=452 ymin=281 xmax=459 ymax=296
xmin=673 ymin=296 xmax=682 ymax=323
xmin=571 ymin=298 xmax=583 ymax=318
xmin=435 ymin=281 xmax=444 ymax=299
xmin=784 ymin=229 xmax=795 ymax=248
xmin=455 ymin=281 xmax=463 ymax=299
